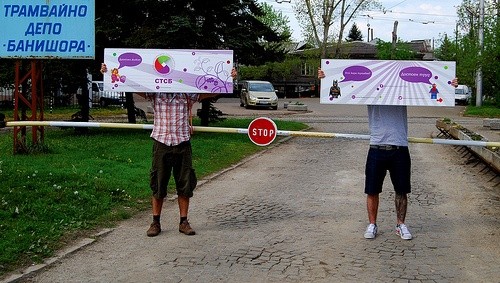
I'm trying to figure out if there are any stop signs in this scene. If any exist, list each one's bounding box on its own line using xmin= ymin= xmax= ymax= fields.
xmin=248 ymin=116 xmax=277 ymax=147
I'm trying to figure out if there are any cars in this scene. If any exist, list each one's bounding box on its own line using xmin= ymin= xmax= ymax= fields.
xmin=454 ymin=84 xmax=471 ymax=106
xmin=91 ymin=80 xmax=127 ymax=108
xmin=240 ymin=80 xmax=279 ymax=110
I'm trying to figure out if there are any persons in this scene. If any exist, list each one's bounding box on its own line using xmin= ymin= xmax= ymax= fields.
xmin=101 ymin=63 xmax=237 ymax=236
xmin=317 ymin=67 xmax=458 ymax=239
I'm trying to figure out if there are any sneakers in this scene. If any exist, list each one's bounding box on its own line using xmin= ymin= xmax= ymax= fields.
xmin=396 ymin=223 xmax=412 ymax=239
xmin=179 ymin=220 xmax=195 ymax=235
xmin=364 ymin=224 xmax=378 ymax=238
xmin=147 ymin=221 xmax=161 ymax=236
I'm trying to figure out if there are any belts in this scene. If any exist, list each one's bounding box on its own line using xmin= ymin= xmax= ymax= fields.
xmin=370 ymin=144 xmax=403 ymax=150
